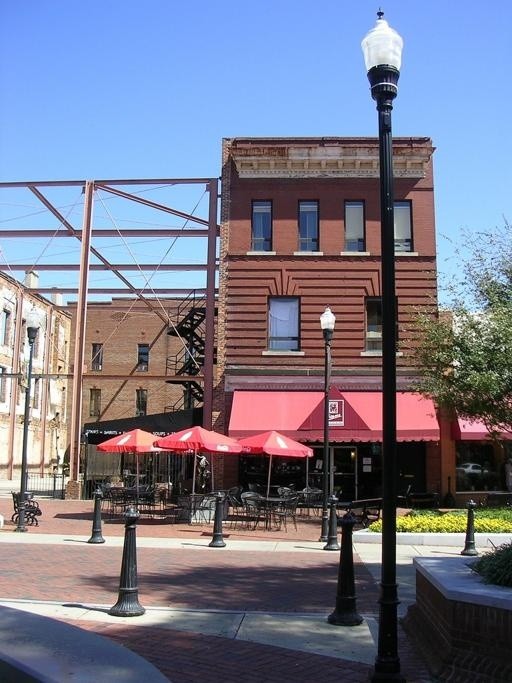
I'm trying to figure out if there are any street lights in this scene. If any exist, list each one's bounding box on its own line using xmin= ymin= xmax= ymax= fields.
xmin=14 ymin=305 xmax=40 ymax=533
xmin=318 ymin=303 xmax=337 ymax=542
xmin=358 ymin=10 xmax=414 ymax=679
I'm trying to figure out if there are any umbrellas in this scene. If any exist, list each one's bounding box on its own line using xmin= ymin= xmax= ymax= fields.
xmin=95 ymin=427 xmax=173 ymax=518
xmin=152 ymin=425 xmax=243 ymax=524
xmin=236 ymin=429 xmax=315 ymax=528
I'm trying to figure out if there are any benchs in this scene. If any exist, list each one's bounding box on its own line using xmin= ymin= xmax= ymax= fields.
xmin=406 ymin=492 xmax=438 ymax=508
xmin=11 ymin=490 xmax=41 ymax=527
xmin=341 ymin=497 xmax=384 ymax=527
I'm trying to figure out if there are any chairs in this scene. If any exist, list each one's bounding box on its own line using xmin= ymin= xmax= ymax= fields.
xmin=95 ymin=482 xmax=338 ymax=529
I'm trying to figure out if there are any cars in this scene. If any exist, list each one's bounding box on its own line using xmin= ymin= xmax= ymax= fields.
xmin=456 ymin=462 xmax=491 ymax=479
